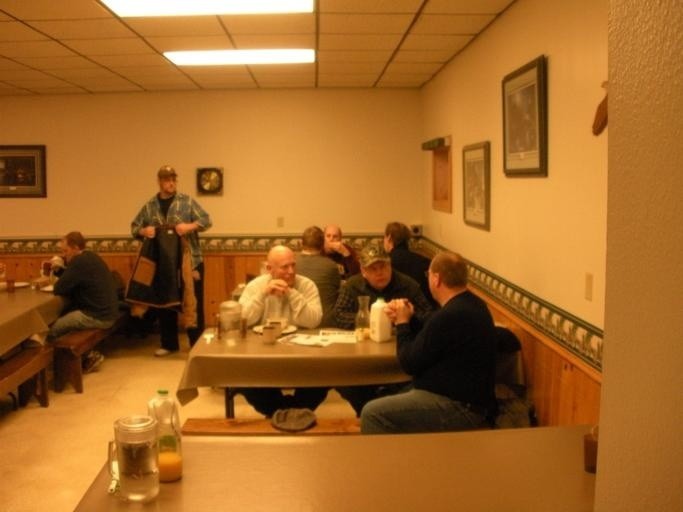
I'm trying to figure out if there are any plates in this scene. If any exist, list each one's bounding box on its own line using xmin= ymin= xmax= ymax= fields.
xmin=252 ymin=324 xmax=296 ymax=334
xmin=0 ymin=281 xmax=29 ymax=288
xmin=41 ymin=285 xmax=53 ymax=292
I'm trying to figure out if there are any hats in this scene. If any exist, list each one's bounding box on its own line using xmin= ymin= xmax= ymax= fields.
xmin=360 ymin=245 xmax=390 ymax=268
xmin=158 ymin=165 xmax=177 ymax=177
xmin=270 ymin=408 xmax=316 ymax=432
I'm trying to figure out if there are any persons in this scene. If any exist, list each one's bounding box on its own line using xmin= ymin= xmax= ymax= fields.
xmin=333 ymin=244 xmax=432 ymax=420
xmin=238 ymin=245 xmax=329 ymax=417
xmin=132 ymin=166 xmax=212 ymax=356
xmin=50 ymin=231 xmax=122 ymax=371
xmin=384 ymin=221 xmax=434 ymax=304
xmin=359 ymin=251 xmax=498 ymax=434
xmin=290 ymin=227 xmax=345 ymax=326
xmin=322 ymin=225 xmax=358 ymax=278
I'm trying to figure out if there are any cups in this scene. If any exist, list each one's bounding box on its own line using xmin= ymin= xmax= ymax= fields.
xmin=5 ymin=277 xmax=15 ymax=294
xmin=42 ymin=261 xmax=52 ymax=275
xmin=216 ymin=299 xmax=244 ymax=345
xmin=259 ymin=320 xmax=281 ymax=346
xmin=31 ymin=280 xmax=40 ymax=292
xmin=581 ymin=432 xmax=599 ymax=475
xmin=107 ymin=416 xmax=159 ymax=505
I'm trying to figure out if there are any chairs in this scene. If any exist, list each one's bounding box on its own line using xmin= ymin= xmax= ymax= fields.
xmin=246 ymin=273 xmax=255 ymax=284
xmin=483 ymin=326 xmax=539 ymax=427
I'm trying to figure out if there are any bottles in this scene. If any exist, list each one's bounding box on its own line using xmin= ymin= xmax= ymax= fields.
xmin=146 ymin=389 xmax=184 ymax=484
xmin=368 ymin=296 xmax=392 ymax=343
xmin=354 ymin=294 xmax=370 ymax=344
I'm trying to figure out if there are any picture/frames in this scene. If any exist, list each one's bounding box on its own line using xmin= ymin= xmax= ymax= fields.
xmin=502 ymin=54 xmax=548 ymax=177
xmin=462 ymin=140 xmax=490 ymax=232
xmin=0 ymin=144 xmax=47 ymax=198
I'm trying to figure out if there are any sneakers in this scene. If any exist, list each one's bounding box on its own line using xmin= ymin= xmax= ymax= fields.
xmin=155 ymin=349 xmax=169 ymax=356
xmin=82 ymin=351 xmax=103 ymax=374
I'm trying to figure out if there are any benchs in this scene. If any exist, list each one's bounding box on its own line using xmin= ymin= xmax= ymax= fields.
xmin=52 ymin=308 xmax=129 ymax=393
xmin=181 ymin=413 xmax=363 ymax=435
xmin=0 ymin=344 xmax=55 ymax=407
